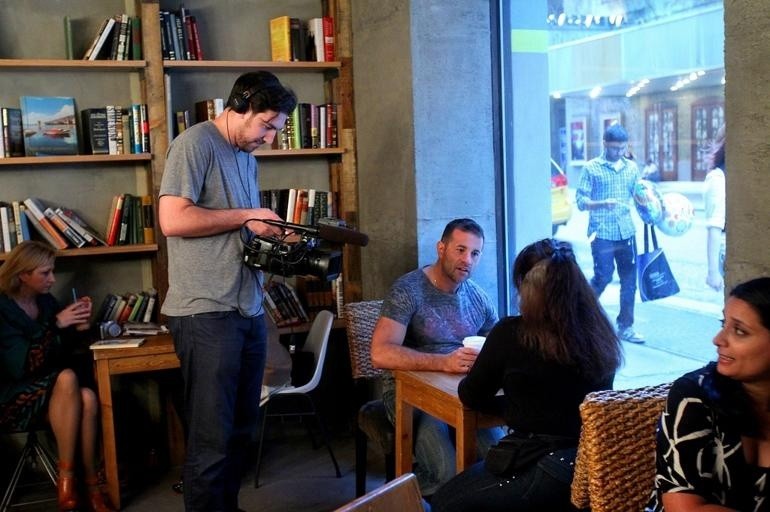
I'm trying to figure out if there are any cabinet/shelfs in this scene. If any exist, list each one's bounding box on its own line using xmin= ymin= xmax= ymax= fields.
xmin=0 ymin=0 xmax=162 ymax=319
xmin=162 ymin=0 xmax=361 ymax=315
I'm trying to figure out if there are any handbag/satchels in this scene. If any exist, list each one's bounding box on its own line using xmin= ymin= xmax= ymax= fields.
xmin=637 ymin=222 xmax=680 ymax=302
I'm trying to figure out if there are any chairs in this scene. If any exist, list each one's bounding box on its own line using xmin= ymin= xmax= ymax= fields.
xmin=572 ymin=383 xmax=679 ymax=512
xmin=336 ymin=472 xmax=425 ymax=511
xmin=247 ymin=309 xmax=343 ymax=488
xmin=580 ymin=396 xmax=671 ymax=511
xmin=347 ymin=300 xmax=396 ymax=498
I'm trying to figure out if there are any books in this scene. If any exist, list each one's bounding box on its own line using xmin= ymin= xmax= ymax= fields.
xmin=1 ymin=92 xmax=150 ymax=160
xmin=0 ymin=193 xmax=155 ymax=249
xmin=269 ymin=14 xmax=336 ymax=62
xmin=270 ymin=101 xmax=338 ymax=150
xmin=89 ymin=286 xmax=157 ymax=351
xmin=260 ymin=274 xmax=345 ymax=328
xmin=158 ymin=2 xmax=203 ymax=61
xmin=162 ymin=72 xmax=224 ymax=145
xmin=58 ymin=13 xmax=143 ymax=60
xmin=257 ymin=187 xmax=335 ymax=228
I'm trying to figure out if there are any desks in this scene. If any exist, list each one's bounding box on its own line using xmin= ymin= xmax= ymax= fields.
xmin=95 ymin=333 xmax=181 ymax=512
xmin=396 ymin=364 xmax=509 ymax=487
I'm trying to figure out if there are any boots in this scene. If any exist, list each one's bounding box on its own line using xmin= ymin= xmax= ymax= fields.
xmin=86 ymin=479 xmax=111 ymax=512
xmin=57 ymin=460 xmax=75 ymax=508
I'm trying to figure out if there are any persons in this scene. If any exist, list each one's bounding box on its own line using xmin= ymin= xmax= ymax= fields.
xmin=642 ymin=158 xmax=661 ymax=182
xmin=702 ymin=121 xmax=727 ymax=291
xmin=576 ymin=122 xmax=650 ymax=344
xmin=0 ymin=240 xmax=111 ymax=512
xmin=370 ymin=218 xmax=500 ymax=495
xmin=431 ymin=238 xmax=622 ymax=511
xmin=157 ymin=69 xmax=297 ymax=511
xmin=642 ymin=276 xmax=770 ymax=512
xmin=623 ymin=145 xmax=638 ymax=161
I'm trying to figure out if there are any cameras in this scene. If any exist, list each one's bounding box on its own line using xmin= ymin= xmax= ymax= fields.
xmin=237 ymin=217 xmax=370 ymax=319
xmin=97 ymin=319 xmax=120 ymax=339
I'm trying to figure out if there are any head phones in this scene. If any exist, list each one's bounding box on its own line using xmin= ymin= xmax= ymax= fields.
xmin=229 ymin=80 xmax=281 ymax=113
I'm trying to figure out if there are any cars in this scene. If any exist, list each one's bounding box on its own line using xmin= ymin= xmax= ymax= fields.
xmin=550 ymin=157 xmax=574 ymax=238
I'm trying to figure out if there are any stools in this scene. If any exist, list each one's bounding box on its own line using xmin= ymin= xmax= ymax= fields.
xmin=0 ymin=418 xmax=80 ymax=511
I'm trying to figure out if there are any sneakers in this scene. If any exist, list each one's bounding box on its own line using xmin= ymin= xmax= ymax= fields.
xmin=618 ymin=325 xmax=645 ymax=343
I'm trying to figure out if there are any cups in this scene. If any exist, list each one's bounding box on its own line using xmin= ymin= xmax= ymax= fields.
xmin=463 ymin=336 xmax=487 ymax=367
xmin=72 ymin=304 xmax=89 ymax=331
